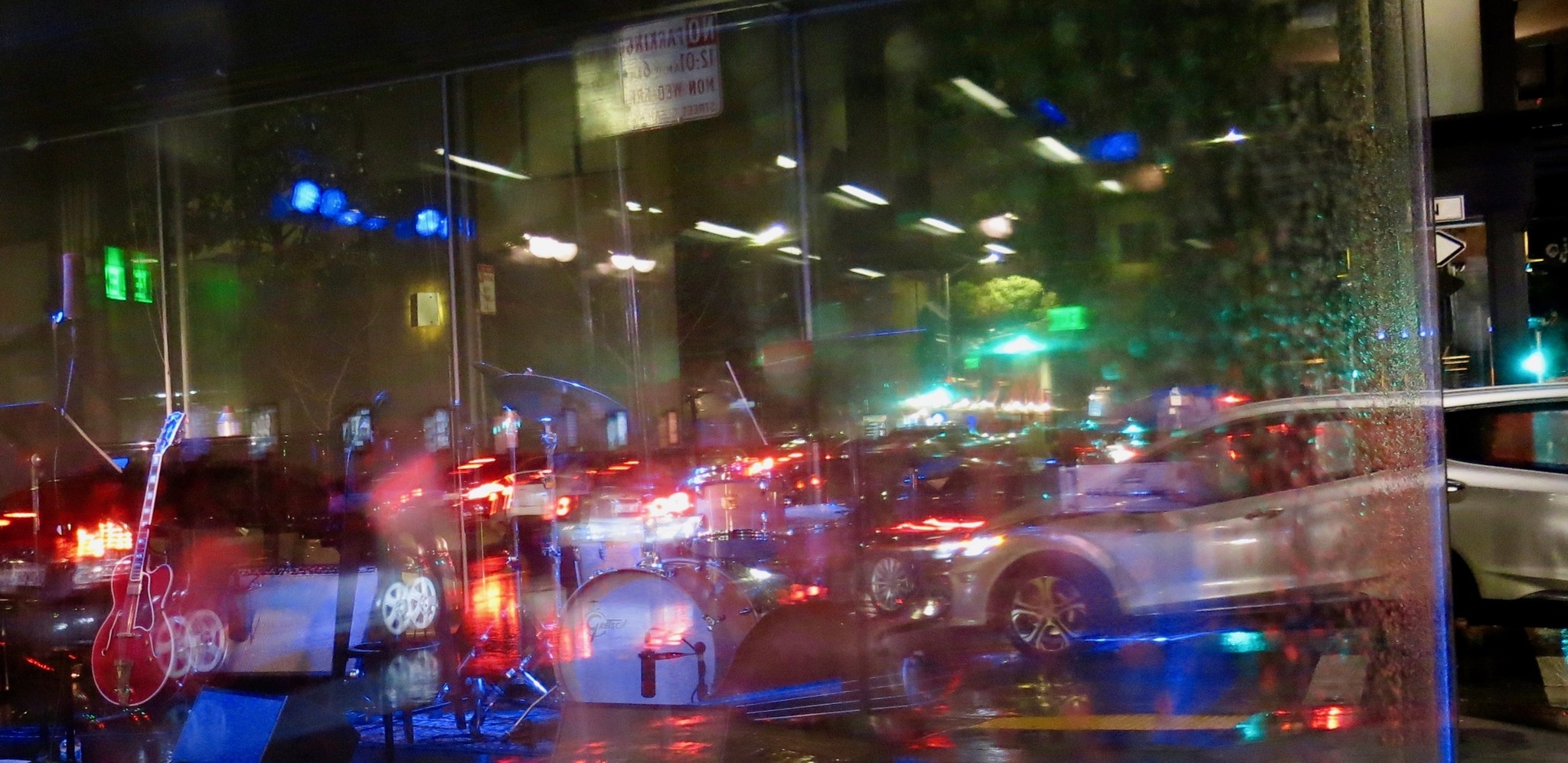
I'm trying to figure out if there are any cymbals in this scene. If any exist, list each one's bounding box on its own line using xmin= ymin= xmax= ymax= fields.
xmin=493 ymin=370 xmax=630 ymax=423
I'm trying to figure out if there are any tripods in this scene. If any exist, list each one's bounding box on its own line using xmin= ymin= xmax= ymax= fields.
xmin=429 ymin=409 xmax=569 ymax=727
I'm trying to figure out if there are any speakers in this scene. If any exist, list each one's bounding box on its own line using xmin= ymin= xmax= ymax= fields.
xmin=172 ymin=565 xmax=385 ymax=763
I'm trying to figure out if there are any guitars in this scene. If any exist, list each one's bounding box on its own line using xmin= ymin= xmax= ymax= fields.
xmin=90 ymin=410 xmax=187 ymax=705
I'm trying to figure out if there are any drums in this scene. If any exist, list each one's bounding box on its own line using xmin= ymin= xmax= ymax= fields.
xmin=570 ymin=516 xmax=687 ymax=582
xmin=687 ymin=465 xmax=789 ymax=561
xmin=776 ymin=500 xmax=855 ymax=573
xmin=552 ymin=557 xmax=759 ymax=704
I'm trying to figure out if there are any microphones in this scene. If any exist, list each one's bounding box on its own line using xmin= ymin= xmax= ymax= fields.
xmin=63 ymin=254 xmax=84 ymax=337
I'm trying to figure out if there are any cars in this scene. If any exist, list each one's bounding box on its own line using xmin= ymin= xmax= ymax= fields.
xmin=944 ymin=385 xmax=1439 ymax=690
xmin=0 ymin=407 xmax=1149 ymax=725
xmin=1442 ymin=378 xmax=1568 ymax=623
